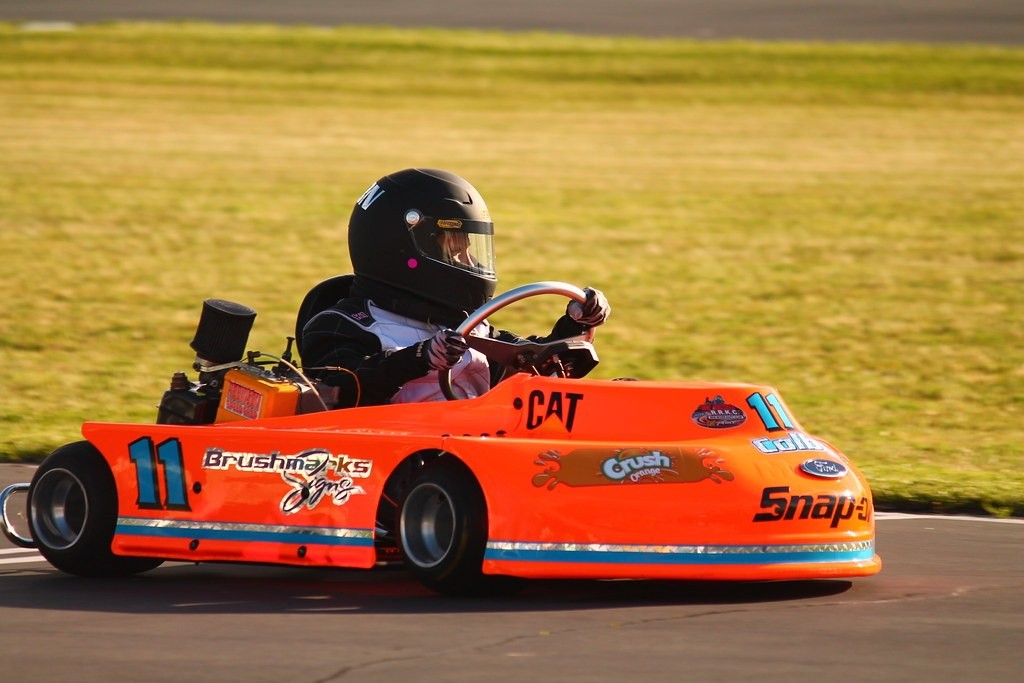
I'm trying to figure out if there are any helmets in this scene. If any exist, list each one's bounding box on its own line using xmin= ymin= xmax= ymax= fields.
xmin=348 ymin=168 xmax=495 ymax=331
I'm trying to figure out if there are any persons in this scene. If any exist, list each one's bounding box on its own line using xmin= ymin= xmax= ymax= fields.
xmin=301 ymin=168 xmax=611 ymax=409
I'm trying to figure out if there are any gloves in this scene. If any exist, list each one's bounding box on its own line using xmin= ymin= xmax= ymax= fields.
xmin=424 ymin=327 xmax=470 ymax=373
